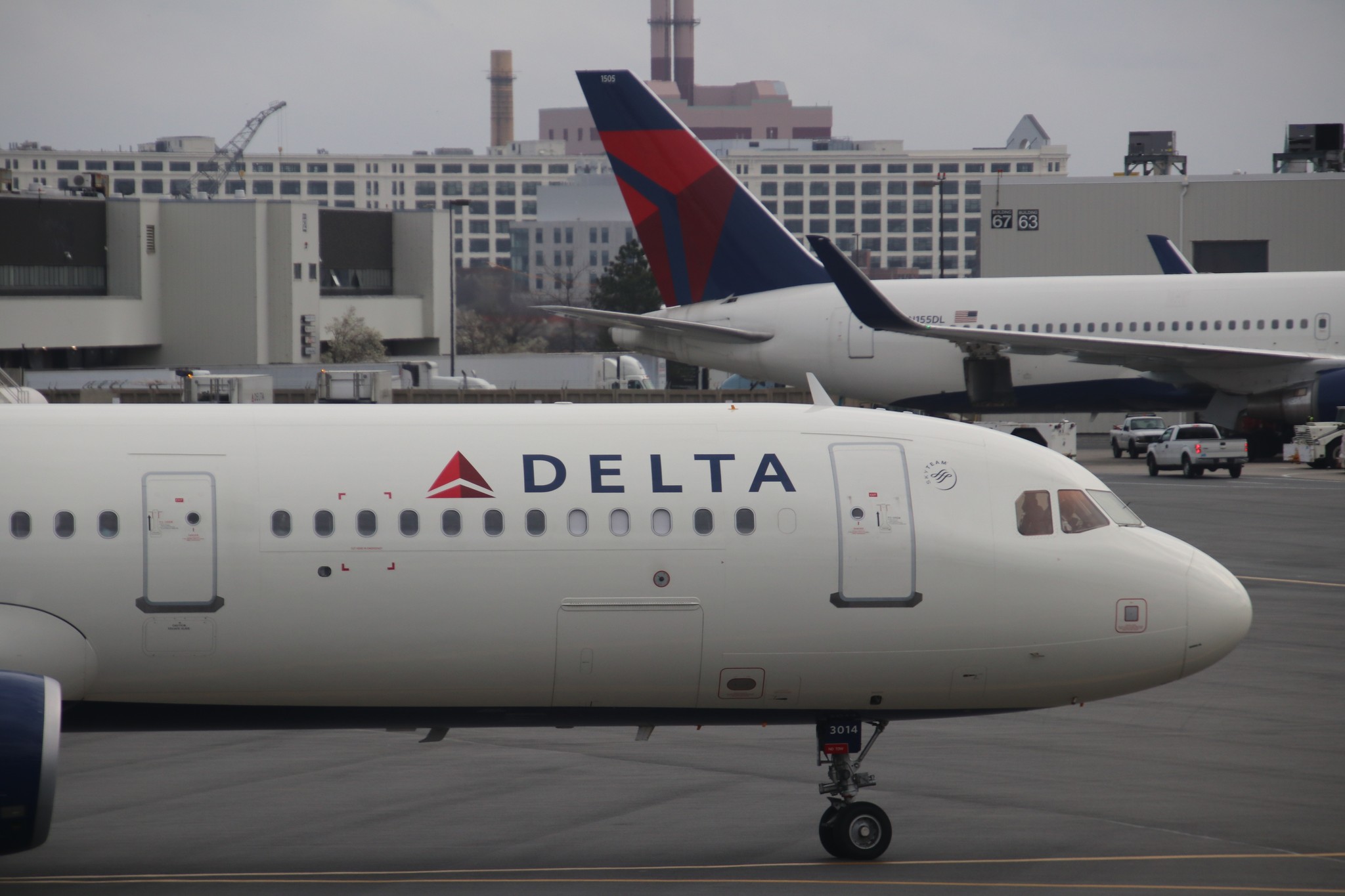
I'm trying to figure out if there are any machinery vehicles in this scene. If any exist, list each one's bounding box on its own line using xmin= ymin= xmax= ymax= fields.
xmin=1282 ymin=406 xmax=1345 ymax=469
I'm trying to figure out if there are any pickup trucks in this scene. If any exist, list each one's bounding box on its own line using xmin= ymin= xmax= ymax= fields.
xmin=1110 ymin=416 xmax=1171 ymax=459
xmin=1147 ymin=423 xmax=1249 ymax=479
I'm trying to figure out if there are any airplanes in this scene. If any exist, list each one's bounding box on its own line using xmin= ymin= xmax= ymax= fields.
xmin=0 ymin=370 xmax=1254 ymax=863
xmin=522 ymin=68 xmax=1345 ymax=426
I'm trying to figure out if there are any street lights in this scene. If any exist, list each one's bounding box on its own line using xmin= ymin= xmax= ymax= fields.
xmin=852 ymin=233 xmax=861 ymax=267
xmin=921 ymin=180 xmax=945 ymax=278
xmin=448 ymin=199 xmax=472 ymax=377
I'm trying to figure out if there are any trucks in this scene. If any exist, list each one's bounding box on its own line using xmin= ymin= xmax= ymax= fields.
xmin=2 ymin=344 xmax=669 ymax=397
